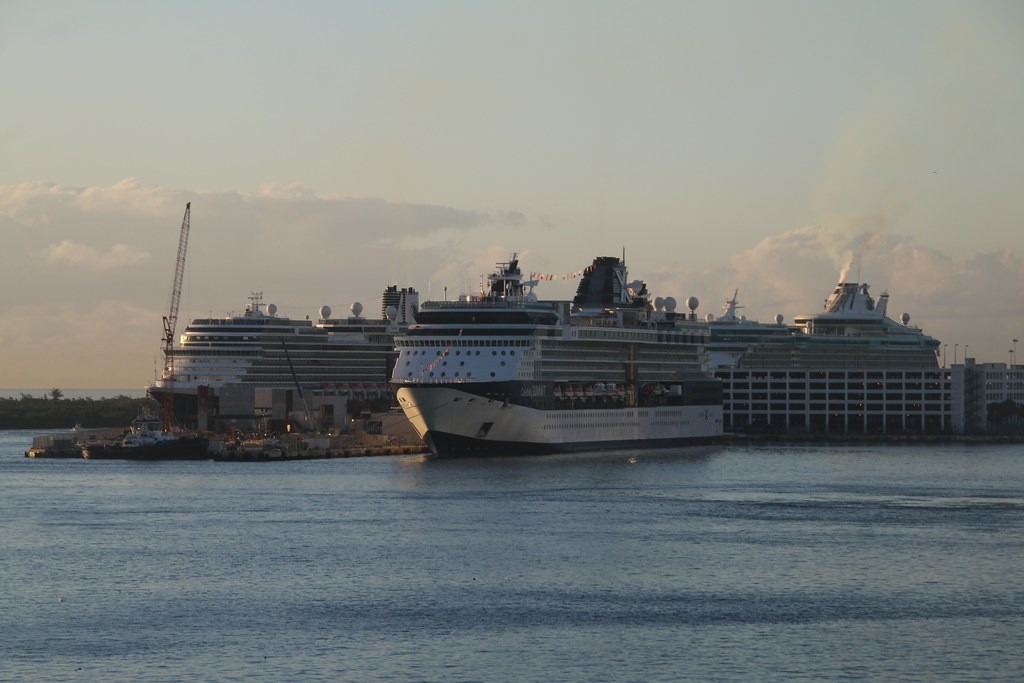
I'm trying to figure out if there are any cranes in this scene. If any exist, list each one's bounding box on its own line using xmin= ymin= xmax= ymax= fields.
xmin=162 ymin=203 xmax=193 ymax=436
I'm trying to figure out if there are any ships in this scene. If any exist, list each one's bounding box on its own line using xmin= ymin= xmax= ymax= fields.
xmin=153 ymin=255 xmax=724 ymax=458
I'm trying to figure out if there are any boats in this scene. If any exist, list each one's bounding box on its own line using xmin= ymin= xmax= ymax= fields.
xmin=80 ymin=414 xmax=207 ymax=460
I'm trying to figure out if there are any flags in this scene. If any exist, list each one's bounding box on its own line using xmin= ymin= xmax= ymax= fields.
xmin=531 ymin=263 xmax=597 ymax=281
xmin=628 ymin=287 xmax=634 ymax=295
